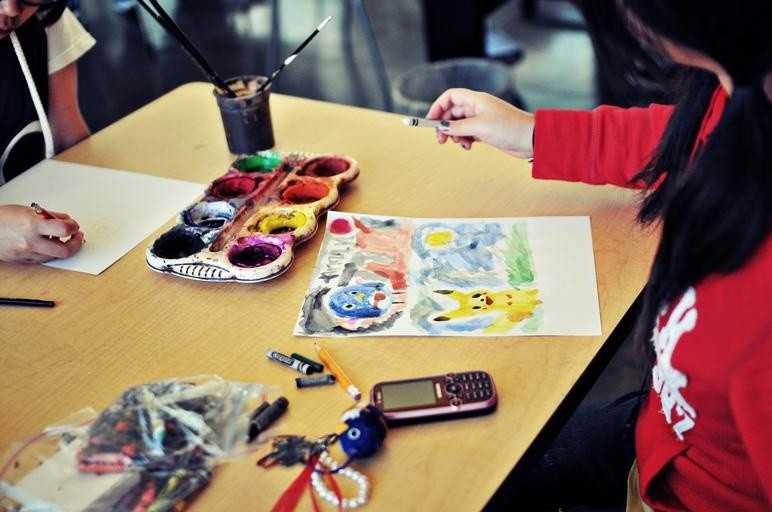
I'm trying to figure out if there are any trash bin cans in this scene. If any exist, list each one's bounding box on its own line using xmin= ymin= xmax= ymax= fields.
xmin=391 ymin=58 xmax=509 ymax=123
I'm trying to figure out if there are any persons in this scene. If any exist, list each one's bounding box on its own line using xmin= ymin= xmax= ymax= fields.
xmin=0 ymin=1 xmax=97 ymax=264
xmin=427 ymin=0 xmax=772 ymax=511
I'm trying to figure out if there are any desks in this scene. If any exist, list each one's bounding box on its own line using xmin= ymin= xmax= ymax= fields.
xmin=2 ymin=75 xmax=682 ymax=512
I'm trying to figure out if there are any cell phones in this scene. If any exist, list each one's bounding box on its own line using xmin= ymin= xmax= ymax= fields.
xmin=370 ymin=370 xmax=497 ymax=428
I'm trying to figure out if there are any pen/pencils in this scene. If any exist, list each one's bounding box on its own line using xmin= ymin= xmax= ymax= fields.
xmin=313 ymin=339 xmax=362 ymax=401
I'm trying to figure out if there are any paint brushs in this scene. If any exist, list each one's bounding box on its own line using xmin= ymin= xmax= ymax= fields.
xmin=78 ymin=383 xmax=236 ymax=476
xmin=114 ymin=471 xmax=214 ymax=511
xmin=404 ymin=118 xmax=443 ymax=128
xmin=1 ymin=297 xmax=56 ymax=309
xmin=31 ymin=201 xmax=85 ymax=243
xmin=290 ymin=350 xmax=328 ymax=373
xmin=246 ymin=16 xmax=331 ymax=91
xmin=247 ymin=401 xmax=269 ymax=420
xmin=268 ymin=347 xmax=313 ymax=375
xmin=138 ymin=1 xmax=228 ymax=101
xmin=152 ymin=2 xmax=237 ymax=101
xmin=243 ymin=396 xmax=289 ymax=443
xmin=296 ymin=376 xmax=336 ymax=387
